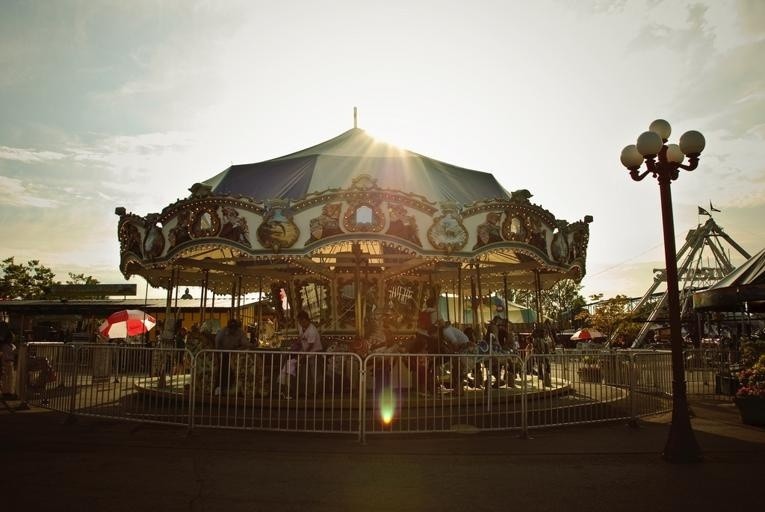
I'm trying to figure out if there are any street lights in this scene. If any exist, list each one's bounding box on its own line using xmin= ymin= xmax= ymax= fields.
xmin=620 ymin=119 xmax=701 ymax=464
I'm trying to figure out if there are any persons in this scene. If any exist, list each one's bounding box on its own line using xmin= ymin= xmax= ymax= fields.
xmin=175 ymin=327 xmax=187 ymax=365
xmin=0 ymin=329 xmax=50 ymax=403
xmin=275 ymin=310 xmax=324 ymax=400
xmin=412 ymin=297 xmax=551 ymax=398
xmin=214 ymin=319 xmax=247 ymax=396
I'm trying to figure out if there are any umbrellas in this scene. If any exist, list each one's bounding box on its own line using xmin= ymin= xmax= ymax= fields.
xmin=569 ymin=328 xmax=608 ymax=366
xmin=99 ymin=309 xmax=156 ymax=371
